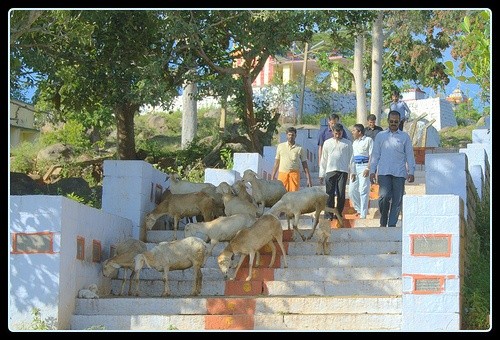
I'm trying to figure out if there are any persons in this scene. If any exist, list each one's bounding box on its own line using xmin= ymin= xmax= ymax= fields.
xmin=364 ymin=114 xmax=383 ymax=185
xmin=318 ymin=113 xmax=349 ymax=196
xmin=318 ymin=123 xmax=356 ymax=221
xmin=349 ymin=124 xmax=374 ymax=219
xmin=271 ymin=126 xmax=312 ymax=194
xmin=369 ymin=111 xmax=415 ymax=227
xmin=390 ymin=91 xmax=411 ymax=132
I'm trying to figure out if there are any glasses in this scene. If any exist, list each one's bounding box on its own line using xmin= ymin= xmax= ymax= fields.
xmin=389 ymin=120 xmax=399 ymax=123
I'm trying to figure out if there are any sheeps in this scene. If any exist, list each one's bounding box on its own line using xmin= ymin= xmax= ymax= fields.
xmin=242 ymin=169 xmax=287 ymax=212
xmin=218 ymin=214 xmax=288 ymax=281
xmin=145 ymin=192 xmax=217 ymax=242
xmin=131 ymin=237 xmax=209 ymax=297
xmin=103 ymin=239 xmax=146 ymax=296
xmin=184 ymin=213 xmax=257 ymax=266
xmin=232 ymin=180 xmax=254 ymax=202
xmin=316 ymin=220 xmax=332 ymax=255
xmin=216 ymin=182 xmax=261 ymax=219
xmin=268 ymin=186 xmax=344 ymax=241
xmin=168 ymin=172 xmax=224 ymax=215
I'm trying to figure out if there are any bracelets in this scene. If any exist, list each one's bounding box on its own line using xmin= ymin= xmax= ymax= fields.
xmin=366 ymin=168 xmax=370 ymax=170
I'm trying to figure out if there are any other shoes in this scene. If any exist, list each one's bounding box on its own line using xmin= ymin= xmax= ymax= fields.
xmin=388 ymin=224 xmax=396 ymax=227
xmin=324 ymin=215 xmax=332 ymax=221
xmin=335 ymin=214 xmax=342 ymax=219
xmin=380 ymin=224 xmax=386 ymax=227
